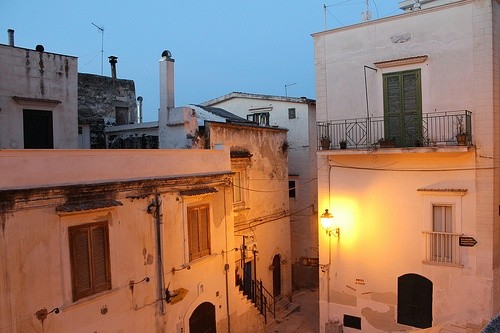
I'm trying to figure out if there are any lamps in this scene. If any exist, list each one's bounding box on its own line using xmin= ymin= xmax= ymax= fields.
xmin=319 ymin=208 xmax=341 ymax=237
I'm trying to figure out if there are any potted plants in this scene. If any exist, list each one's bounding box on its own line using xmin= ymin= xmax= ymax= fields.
xmin=416 ymin=137 xmax=423 ymax=147
xmin=319 ymin=133 xmax=331 ymax=150
xmin=455 ymin=115 xmax=470 ymax=145
xmin=377 ymin=137 xmax=396 ymax=148
xmin=338 ymin=138 xmax=347 ymax=149
